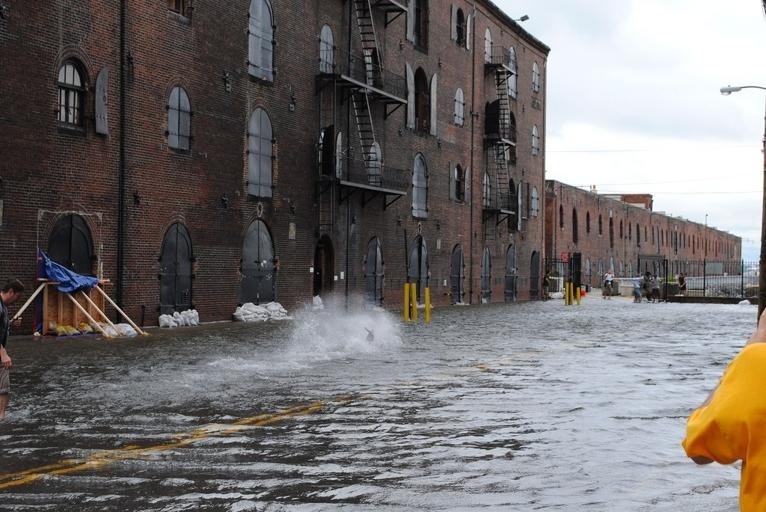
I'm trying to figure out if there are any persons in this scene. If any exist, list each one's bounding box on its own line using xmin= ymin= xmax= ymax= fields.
xmin=603 ymin=269 xmax=615 ymax=300
xmin=633 ymin=271 xmax=663 ymax=304
xmin=681 ymin=305 xmax=766 ymax=512
xmin=0 ymin=279 xmax=24 ymax=419
xmin=544 ymin=269 xmax=551 ymax=301
xmin=678 ymin=272 xmax=686 ymax=294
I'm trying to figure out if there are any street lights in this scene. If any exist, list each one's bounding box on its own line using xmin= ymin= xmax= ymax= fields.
xmin=719 ymin=84 xmax=766 ymax=329
